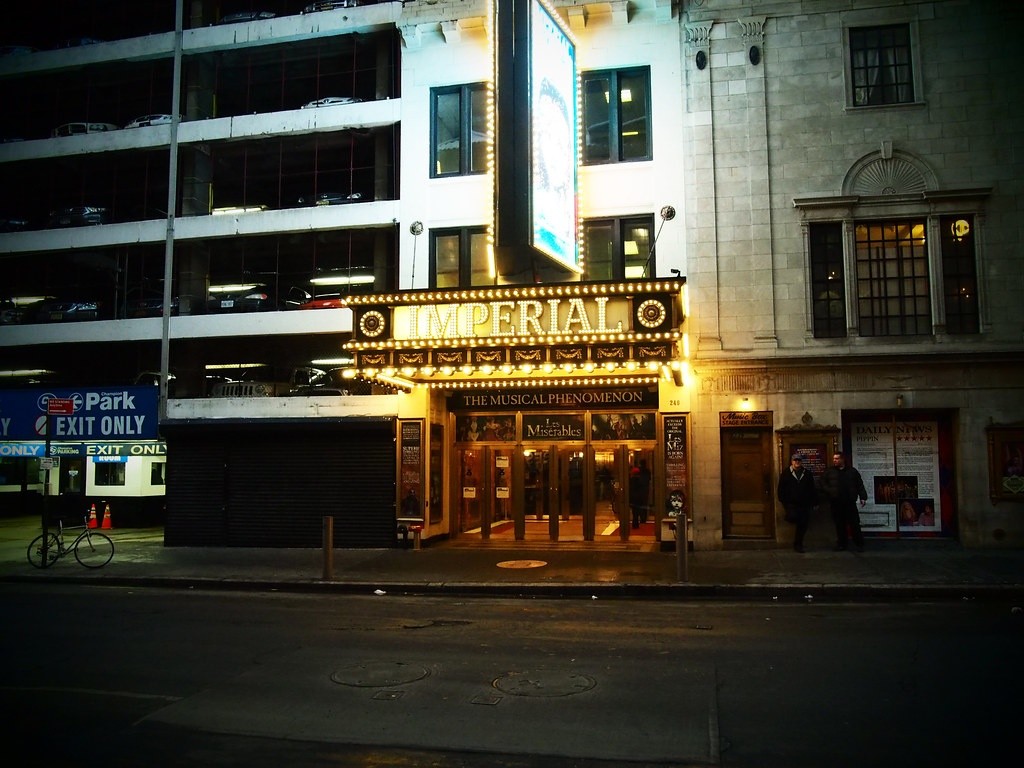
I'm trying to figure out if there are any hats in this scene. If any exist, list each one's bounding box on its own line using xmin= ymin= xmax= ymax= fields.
xmin=792 ymin=454 xmax=802 ymax=460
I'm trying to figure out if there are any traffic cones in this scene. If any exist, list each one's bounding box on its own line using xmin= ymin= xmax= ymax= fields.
xmin=87 ymin=502 xmax=100 ymax=530
xmin=100 ymin=504 xmax=116 ymax=530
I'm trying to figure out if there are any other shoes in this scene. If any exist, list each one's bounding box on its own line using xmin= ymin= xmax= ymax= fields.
xmin=795 ymin=548 xmax=805 ymax=553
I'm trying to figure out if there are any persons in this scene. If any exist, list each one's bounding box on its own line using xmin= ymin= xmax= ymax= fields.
xmin=629 ymin=459 xmax=651 ymax=528
xmin=918 ymin=504 xmax=934 ymax=526
xmin=818 ymin=451 xmax=868 ymax=553
xmin=403 ymin=489 xmax=419 ymax=516
xmin=1005 ymin=456 xmax=1023 ymax=476
xmin=667 ymin=489 xmax=685 ymax=517
xmin=776 ymin=453 xmax=819 ymax=553
xmin=899 ymin=501 xmax=923 ymax=526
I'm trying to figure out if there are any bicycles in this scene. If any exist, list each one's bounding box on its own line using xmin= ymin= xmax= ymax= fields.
xmin=27 ymin=509 xmax=114 ymax=569
